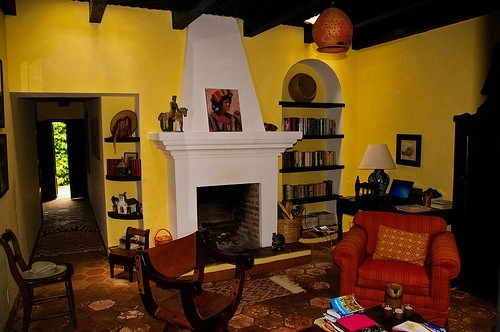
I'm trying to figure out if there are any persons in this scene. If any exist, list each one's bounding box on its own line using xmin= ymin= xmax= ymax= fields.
xmin=170 ymin=95 xmax=179 ymax=122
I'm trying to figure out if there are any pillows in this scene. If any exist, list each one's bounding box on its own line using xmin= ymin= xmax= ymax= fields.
xmin=371 ymin=223 xmax=430 ymax=267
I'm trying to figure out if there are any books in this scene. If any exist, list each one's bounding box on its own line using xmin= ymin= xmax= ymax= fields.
xmin=282 ymin=180 xmax=334 ymax=199
xmin=395 ymin=204 xmax=432 ymax=213
xmin=282 ymin=118 xmax=336 ymax=136
xmin=314 ymin=293 xmax=384 ymax=332
xmin=431 ymin=199 xmax=452 ymax=210
xmin=380 ymin=319 xmax=446 ymax=332
xmin=282 ymin=150 xmax=336 ymax=168
xmin=300 ymin=211 xmax=336 ymax=229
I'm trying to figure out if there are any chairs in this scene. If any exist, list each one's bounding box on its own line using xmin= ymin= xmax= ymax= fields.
xmin=130 ymin=231 xmax=256 ymax=332
xmin=354 ymin=176 xmax=380 ymax=213
xmin=0 ymin=228 xmax=78 ymax=332
xmin=107 ymin=226 xmax=152 ymax=282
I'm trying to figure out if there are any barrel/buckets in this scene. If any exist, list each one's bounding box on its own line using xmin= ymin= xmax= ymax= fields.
xmin=153 ymin=228 xmax=172 ymax=247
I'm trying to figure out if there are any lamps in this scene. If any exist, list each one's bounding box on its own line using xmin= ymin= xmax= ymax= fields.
xmin=357 ymin=142 xmax=398 ymax=195
xmin=311 ymin=3 xmax=354 ymax=54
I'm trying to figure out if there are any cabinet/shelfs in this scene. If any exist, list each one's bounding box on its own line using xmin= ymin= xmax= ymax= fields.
xmin=277 ymin=99 xmax=346 ymax=204
xmin=104 ymin=137 xmax=143 ymax=221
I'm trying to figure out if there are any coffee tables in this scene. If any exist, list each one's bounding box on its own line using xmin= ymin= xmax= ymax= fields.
xmin=295 ymin=303 xmax=451 ymax=332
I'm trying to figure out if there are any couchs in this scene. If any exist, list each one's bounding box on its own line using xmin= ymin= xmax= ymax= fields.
xmin=333 ymin=209 xmax=463 ymax=326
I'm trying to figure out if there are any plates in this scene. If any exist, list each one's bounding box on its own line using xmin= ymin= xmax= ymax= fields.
xmin=109 ymin=109 xmax=137 ymax=137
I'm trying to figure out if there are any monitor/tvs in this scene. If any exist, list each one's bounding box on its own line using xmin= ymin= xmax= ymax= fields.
xmin=388 ymin=178 xmax=414 ymax=203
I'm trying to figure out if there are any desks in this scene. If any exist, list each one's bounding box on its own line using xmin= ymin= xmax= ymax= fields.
xmin=336 ymin=197 xmax=452 ymax=243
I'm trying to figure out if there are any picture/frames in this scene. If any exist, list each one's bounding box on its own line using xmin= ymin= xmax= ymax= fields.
xmin=0 ymin=59 xmax=9 ymax=198
xmin=394 ymin=132 xmax=422 ymax=169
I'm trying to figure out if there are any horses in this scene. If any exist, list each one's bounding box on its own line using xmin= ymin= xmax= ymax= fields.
xmin=158 ymin=107 xmax=188 ymax=132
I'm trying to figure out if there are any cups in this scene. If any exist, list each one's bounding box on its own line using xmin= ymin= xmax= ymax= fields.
xmin=423 ymin=194 xmax=430 ymax=207
xmin=383 ymin=304 xmax=414 ymax=320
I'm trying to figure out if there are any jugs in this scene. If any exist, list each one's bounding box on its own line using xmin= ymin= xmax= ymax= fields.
xmin=384 ymin=283 xmax=402 ymax=315
